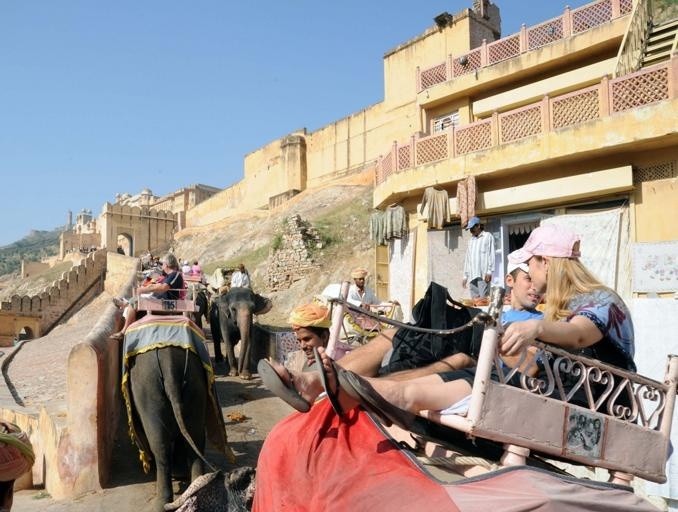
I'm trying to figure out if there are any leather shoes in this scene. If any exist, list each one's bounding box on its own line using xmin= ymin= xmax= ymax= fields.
xmin=337 ymin=368 xmax=416 ymax=432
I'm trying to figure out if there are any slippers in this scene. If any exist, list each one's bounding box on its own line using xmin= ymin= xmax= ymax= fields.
xmin=258 ymin=359 xmax=312 ymax=413
xmin=109 ymin=332 xmax=124 ymax=340
xmin=313 ymin=347 xmax=345 ymax=419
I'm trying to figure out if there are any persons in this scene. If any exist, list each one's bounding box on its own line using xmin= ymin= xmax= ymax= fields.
xmin=109 ymin=253 xmax=250 ymax=340
xmin=0 ymin=420 xmax=34 ymax=512
xmin=462 ymin=217 xmax=495 ymax=300
xmin=288 ymin=303 xmax=345 ymax=372
xmin=257 ymin=260 xmax=544 ymax=418
xmin=346 ymin=268 xmax=400 ymax=311
xmin=338 ymin=222 xmax=635 ymax=430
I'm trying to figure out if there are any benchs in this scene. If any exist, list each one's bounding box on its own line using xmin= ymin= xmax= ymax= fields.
xmin=323 ymin=281 xmax=677 ymax=486
xmin=136 ymin=281 xmax=200 ymax=316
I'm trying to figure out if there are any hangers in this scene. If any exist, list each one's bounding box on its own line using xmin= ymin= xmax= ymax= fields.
xmin=433 ymin=180 xmax=444 ymax=191
xmin=389 ymin=197 xmax=400 ymax=208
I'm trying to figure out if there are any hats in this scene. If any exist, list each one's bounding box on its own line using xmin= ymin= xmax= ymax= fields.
xmin=507 ymin=247 xmax=530 ymax=275
xmin=507 ymin=223 xmax=581 ymax=265
xmin=465 ymin=217 xmax=480 ymax=229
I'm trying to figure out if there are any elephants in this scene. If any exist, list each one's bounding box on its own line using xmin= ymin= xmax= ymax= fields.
xmin=209 ymin=286 xmax=272 ymax=380
xmin=127 ymin=343 xmax=209 ymax=512
